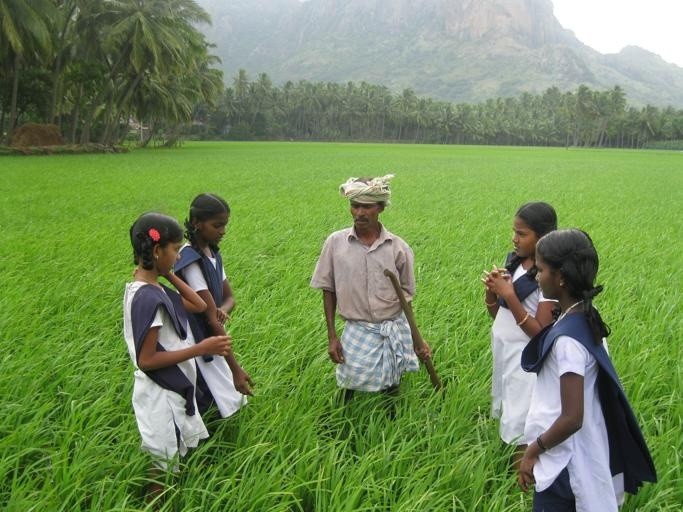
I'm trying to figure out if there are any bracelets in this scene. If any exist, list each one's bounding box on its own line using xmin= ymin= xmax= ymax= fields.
xmin=484 ymin=298 xmax=496 ymax=307
xmin=516 ymin=313 xmax=530 ymax=326
xmin=537 ymin=437 xmax=547 ymax=451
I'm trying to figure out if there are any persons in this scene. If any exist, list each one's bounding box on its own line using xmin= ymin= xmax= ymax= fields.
xmin=173 ymin=193 xmax=256 ymax=419
xmin=124 ymin=211 xmax=233 ymax=512
xmin=481 ymin=201 xmax=557 ymax=471
xmin=310 ymin=174 xmax=431 ymax=421
xmin=518 ymin=228 xmax=657 ymax=511
